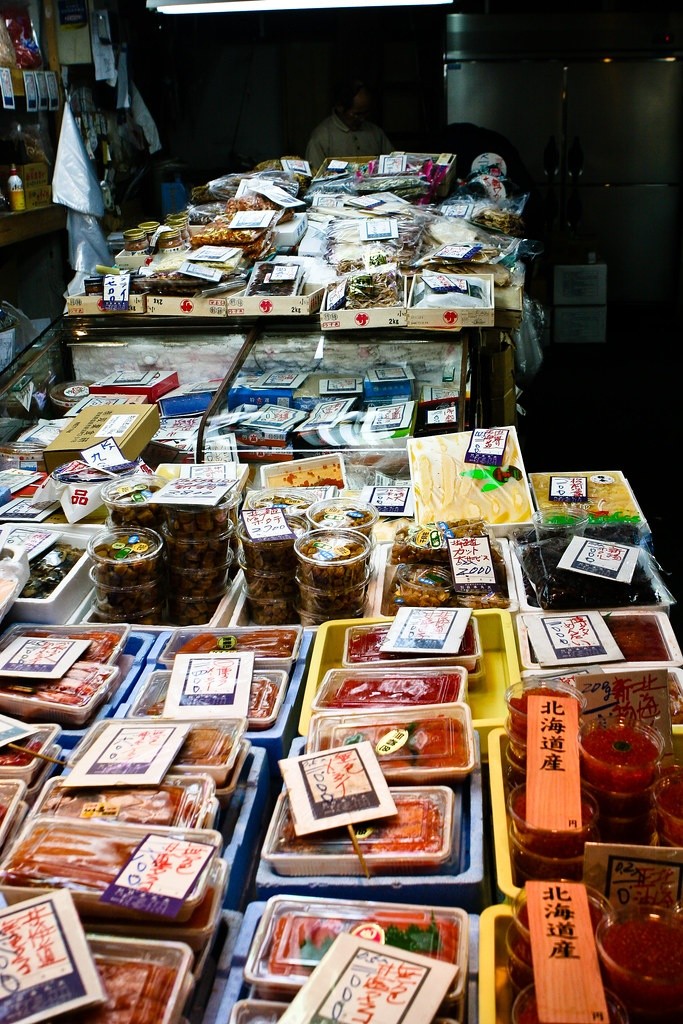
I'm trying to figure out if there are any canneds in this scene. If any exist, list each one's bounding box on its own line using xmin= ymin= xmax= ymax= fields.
xmin=122 ymin=214 xmax=190 ymax=256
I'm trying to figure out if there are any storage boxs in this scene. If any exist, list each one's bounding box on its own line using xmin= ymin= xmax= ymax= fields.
xmin=0 ymin=162 xmax=52 ymax=210
xmin=0 ymin=153 xmax=683 ymax=1024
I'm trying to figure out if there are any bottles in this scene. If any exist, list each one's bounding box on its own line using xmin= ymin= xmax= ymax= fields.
xmin=84 ymin=274 xmax=145 ymax=297
xmin=123 ymin=213 xmax=191 ymax=256
xmin=7 ymin=163 xmax=27 ymax=211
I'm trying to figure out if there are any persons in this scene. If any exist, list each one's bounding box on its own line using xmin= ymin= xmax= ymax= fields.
xmin=306 ymin=85 xmax=395 ymax=171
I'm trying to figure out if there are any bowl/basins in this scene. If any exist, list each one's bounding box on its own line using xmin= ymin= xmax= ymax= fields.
xmin=0 ymin=440 xmax=47 ymax=464
xmin=50 ymin=380 xmax=98 ymax=413
xmin=500 ymin=677 xmax=683 ymax=1024
xmin=531 ymin=505 xmax=591 ymax=542
xmin=395 ymin=563 xmax=454 ymax=608
xmin=0 ymin=620 xmax=489 ymax=1023
xmin=89 ymin=474 xmax=382 ymax=626
